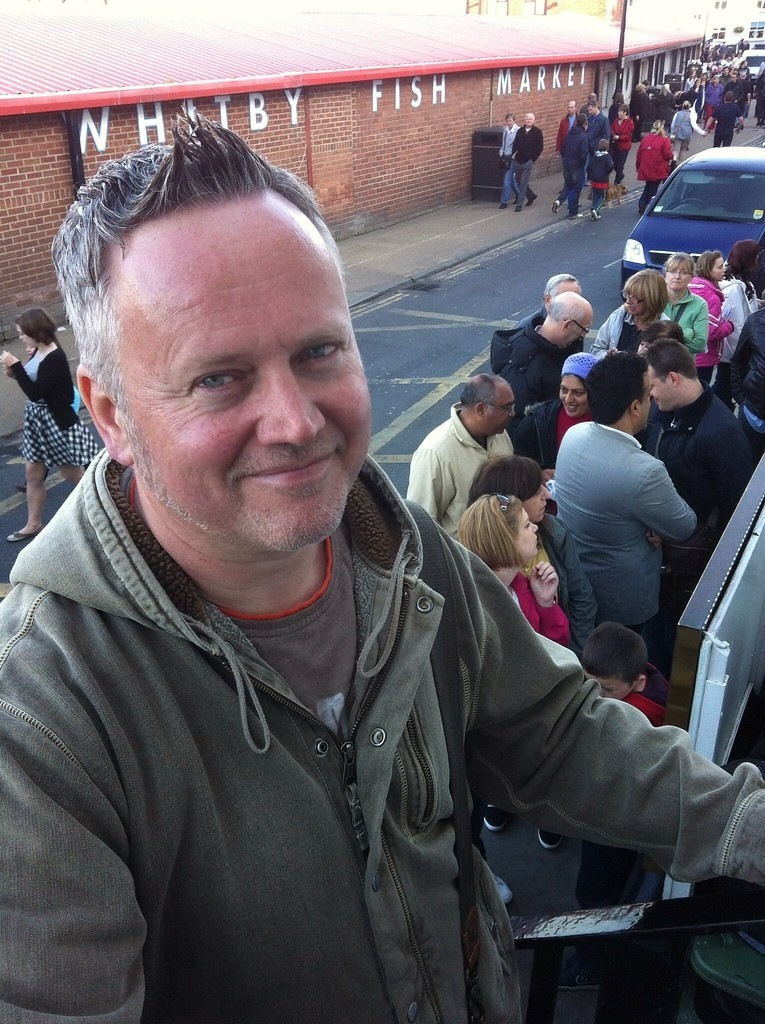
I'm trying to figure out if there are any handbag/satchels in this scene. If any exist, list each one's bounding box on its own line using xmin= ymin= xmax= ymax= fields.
xmin=669 ymin=135 xmax=675 ymax=143
xmin=499 ymin=155 xmax=511 ymax=170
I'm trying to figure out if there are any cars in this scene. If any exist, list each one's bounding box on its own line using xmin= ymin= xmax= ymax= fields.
xmin=620 ymin=146 xmax=765 ymax=303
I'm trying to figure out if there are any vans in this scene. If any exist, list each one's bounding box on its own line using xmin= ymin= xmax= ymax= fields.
xmin=742 ymin=49 xmax=765 ymax=81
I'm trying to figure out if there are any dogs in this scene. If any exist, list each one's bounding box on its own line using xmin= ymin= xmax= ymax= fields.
xmin=603 ymin=184 xmax=630 ymax=208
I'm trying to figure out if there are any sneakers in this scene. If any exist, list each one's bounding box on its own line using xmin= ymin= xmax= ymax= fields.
xmin=492 ymin=870 xmax=512 ymax=905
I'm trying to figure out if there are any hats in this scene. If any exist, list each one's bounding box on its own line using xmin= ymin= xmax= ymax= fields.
xmin=561 ymin=351 xmax=599 ymax=379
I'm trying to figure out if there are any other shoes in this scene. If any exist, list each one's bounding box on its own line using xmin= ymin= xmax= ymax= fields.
xmin=551 ymin=200 xmax=559 ymax=214
xmin=514 ymin=206 xmax=521 ymax=212
xmin=512 ymin=197 xmax=517 ymax=204
xmin=568 ymin=213 xmax=583 ymax=219
xmin=536 ymin=828 xmax=562 ymax=849
xmin=525 ymin=195 xmax=536 ymax=206
xmin=590 ymin=210 xmax=601 ymax=221
xmin=499 ymin=203 xmax=506 ymax=209
xmin=483 ymin=802 xmax=505 ymax=832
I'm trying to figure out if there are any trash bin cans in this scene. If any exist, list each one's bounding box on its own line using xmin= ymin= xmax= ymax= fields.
xmin=664 ymin=73 xmax=685 ymax=93
xmin=641 ymin=88 xmax=660 ymax=132
xmin=673 ymin=933 xmax=765 ymax=1024
xmin=472 ymin=125 xmax=517 ymax=202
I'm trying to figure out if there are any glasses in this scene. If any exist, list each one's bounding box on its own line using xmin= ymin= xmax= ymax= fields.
xmin=621 ymin=291 xmax=645 ymax=304
xmin=563 ymin=319 xmax=588 ymax=335
xmin=666 ymin=270 xmax=692 ymax=276
xmin=490 ymin=403 xmax=514 ymax=413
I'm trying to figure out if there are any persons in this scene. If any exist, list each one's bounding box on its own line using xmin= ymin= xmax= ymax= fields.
xmin=405 ymin=240 xmax=765 ymax=990
xmin=497 ymin=37 xmax=765 ymax=219
xmin=0 ymin=111 xmax=765 ymax=1024
xmin=0 ymin=307 xmax=101 ymax=542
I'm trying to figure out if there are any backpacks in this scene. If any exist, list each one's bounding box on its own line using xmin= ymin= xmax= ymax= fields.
xmin=663 ymin=153 xmax=677 ymax=184
